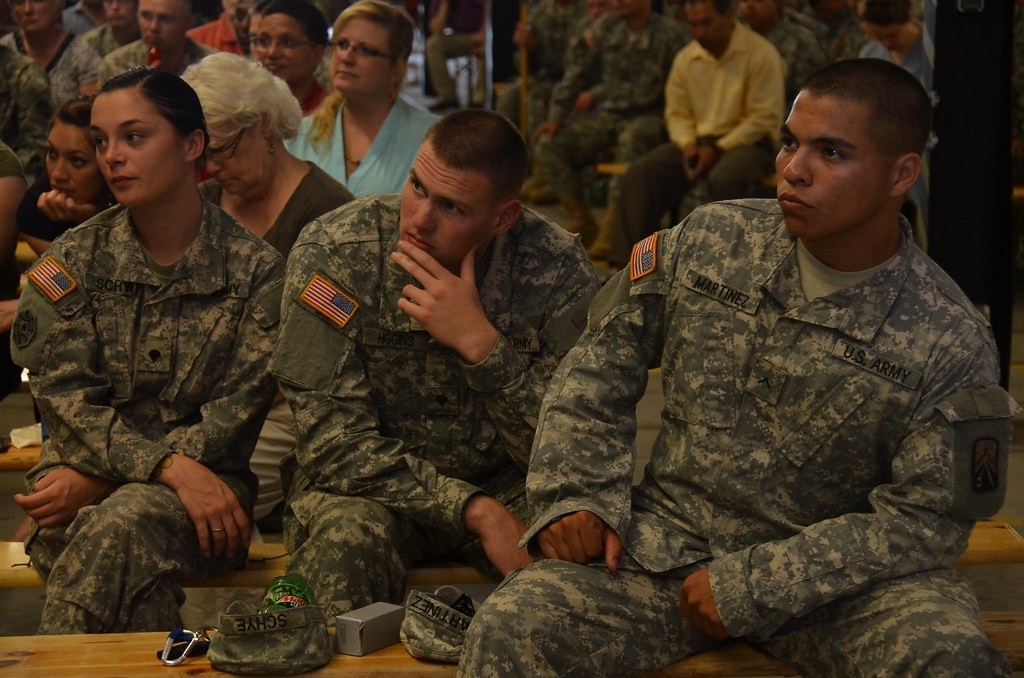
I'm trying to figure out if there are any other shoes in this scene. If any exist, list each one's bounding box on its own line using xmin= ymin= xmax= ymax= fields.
xmin=566 ymin=208 xmax=599 ymax=244
xmin=429 ymin=98 xmax=459 ymax=112
xmin=590 ymin=204 xmax=626 ymax=258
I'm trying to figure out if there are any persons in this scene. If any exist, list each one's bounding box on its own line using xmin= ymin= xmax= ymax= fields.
xmin=266 ymin=110 xmax=602 ymax=627
xmin=1 ymin=0 xmax=337 ymax=191
xmin=180 ymin=52 xmax=357 ymax=260
xmin=0 ymin=140 xmax=29 ymax=303
xmin=424 ymin=0 xmax=935 ymax=287
xmin=454 ymin=58 xmax=1024 ymax=678
xmin=0 ymin=94 xmax=118 ymax=333
xmin=10 ymin=68 xmax=286 ymax=636
xmin=282 ymin=0 xmax=446 ymax=200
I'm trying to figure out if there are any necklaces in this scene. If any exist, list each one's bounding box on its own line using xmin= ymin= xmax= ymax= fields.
xmin=344 ymin=153 xmax=361 ymax=166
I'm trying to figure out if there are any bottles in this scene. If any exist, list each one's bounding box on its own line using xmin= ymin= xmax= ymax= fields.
xmin=259 ymin=575 xmax=316 ymax=617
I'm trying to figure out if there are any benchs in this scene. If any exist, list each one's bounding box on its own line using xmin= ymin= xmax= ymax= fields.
xmin=596 ymin=162 xmax=779 ymax=192
xmin=0 ymin=449 xmax=1024 ymax=678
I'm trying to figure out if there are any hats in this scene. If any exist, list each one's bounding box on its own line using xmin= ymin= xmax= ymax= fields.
xmin=400 ymin=585 xmax=485 ymax=663
xmin=205 ymin=604 xmax=330 ymax=675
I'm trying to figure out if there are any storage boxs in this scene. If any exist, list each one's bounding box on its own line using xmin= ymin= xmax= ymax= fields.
xmin=335 ymin=602 xmax=404 ymax=657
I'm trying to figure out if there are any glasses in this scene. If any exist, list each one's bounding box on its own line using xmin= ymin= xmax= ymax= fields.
xmin=134 ymin=11 xmax=191 ymax=25
xmin=196 ymin=127 xmax=247 ymax=166
xmin=257 ymin=35 xmax=320 ymax=49
xmin=329 ymin=37 xmax=394 ymax=63
xmin=448 ymin=592 xmax=476 ymax=618
